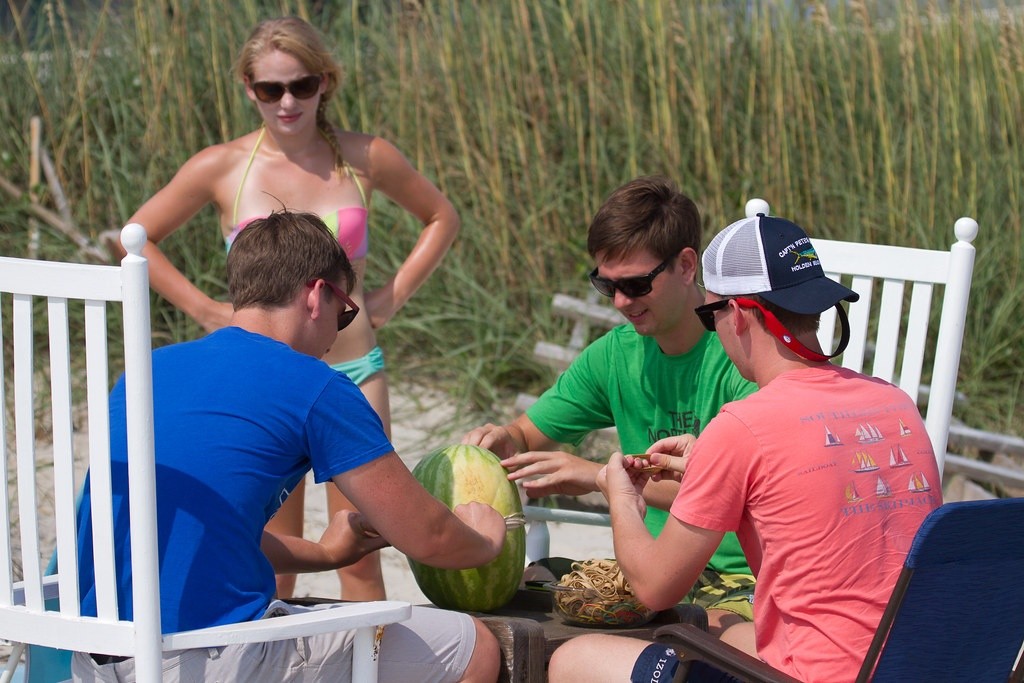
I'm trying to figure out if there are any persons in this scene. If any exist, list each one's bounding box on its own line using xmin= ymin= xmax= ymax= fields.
xmin=79 ymin=212 xmax=508 ymax=683
xmin=118 ymin=16 xmax=461 ymax=602
xmin=461 ymin=174 xmax=760 ymax=641
xmin=548 ymin=213 xmax=943 ymax=683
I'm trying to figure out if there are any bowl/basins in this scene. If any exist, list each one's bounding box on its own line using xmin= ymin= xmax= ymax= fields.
xmin=543 ymin=580 xmax=659 ymax=628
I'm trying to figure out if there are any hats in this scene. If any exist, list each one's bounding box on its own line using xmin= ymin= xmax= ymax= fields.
xmin=699 ymin=213 xmax=861 ymax=319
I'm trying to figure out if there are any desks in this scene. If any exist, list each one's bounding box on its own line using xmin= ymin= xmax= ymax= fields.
xmin=418 ymin=602 xmax=710 ymax=683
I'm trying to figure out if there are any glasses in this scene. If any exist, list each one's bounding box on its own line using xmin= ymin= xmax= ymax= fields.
xmin=588 ymin=258 xmax=669 ymax=299
xmin=306 ymin=277 xmax=360 ymax=333
xmin=695 ymin=298 xmax=764 ymax=331
xmin=245 ymin=75 xmax=325 ymax=104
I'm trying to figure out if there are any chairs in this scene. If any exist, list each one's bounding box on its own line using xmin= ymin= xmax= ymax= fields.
xmin=522 ymin=199 xmax=979 ymax=563
xmin=653 ymin=496 xmax=1023 ymax=683
xmin=0 ymin=223 xmax=412 ymax=683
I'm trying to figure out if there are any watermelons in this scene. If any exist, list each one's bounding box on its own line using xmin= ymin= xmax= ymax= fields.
xmin=411 ymin=444 xmax=532 ymax=612
